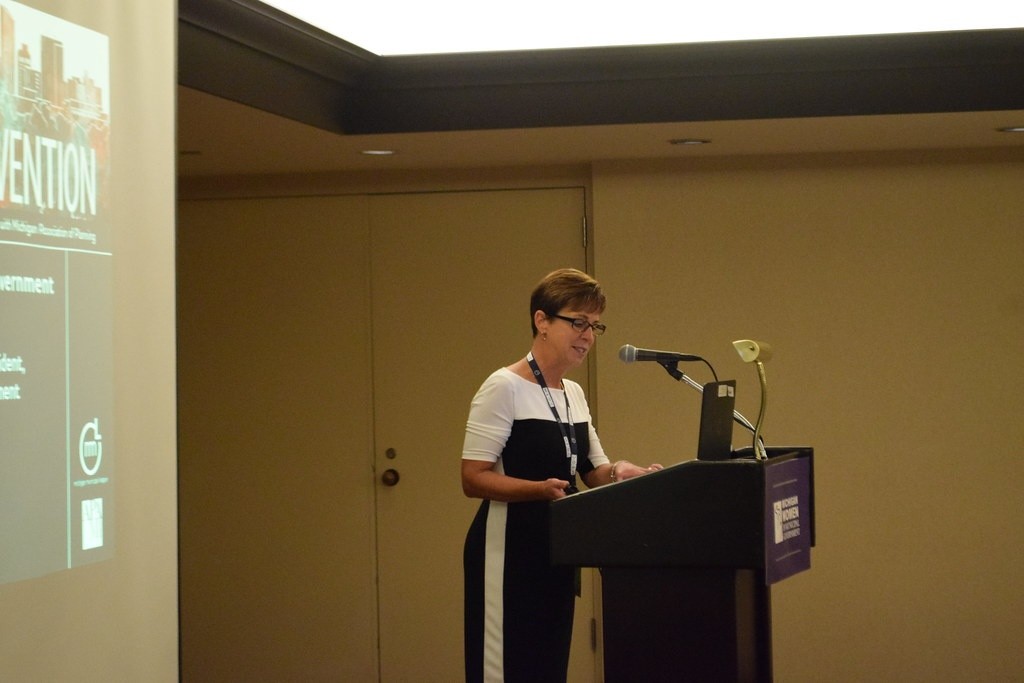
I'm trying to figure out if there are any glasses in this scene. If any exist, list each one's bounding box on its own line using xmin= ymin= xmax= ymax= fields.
xmin=543 ymin=312 xmax=605 ymax=337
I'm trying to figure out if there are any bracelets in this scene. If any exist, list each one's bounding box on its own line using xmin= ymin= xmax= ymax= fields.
xmin=611 ymin=460 xmax=629 ymax=483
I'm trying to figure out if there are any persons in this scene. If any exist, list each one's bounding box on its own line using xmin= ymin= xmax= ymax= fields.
xmin=461 ymin=268 xmax=663 ymax=683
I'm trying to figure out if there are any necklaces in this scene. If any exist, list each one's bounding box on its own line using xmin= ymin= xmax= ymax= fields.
xmin=526 ymin=351 xmax=577 ymax=489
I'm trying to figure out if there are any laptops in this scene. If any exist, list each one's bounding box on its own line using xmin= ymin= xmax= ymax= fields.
xmin=697 ymin=380 xmax=737 ymax=460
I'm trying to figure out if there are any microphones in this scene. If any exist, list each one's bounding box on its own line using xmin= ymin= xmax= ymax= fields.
xmin=618 ymin=344 xmax=702 ymax=363
